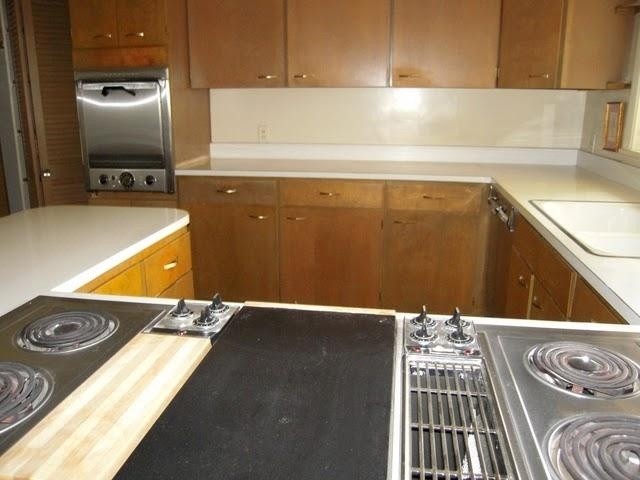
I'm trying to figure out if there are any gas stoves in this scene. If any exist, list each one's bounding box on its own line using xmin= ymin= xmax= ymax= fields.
xmin=401 ymin=304 xmax=639 ymax=480
xmin=1 ymin=294 xmax=233 ymax=458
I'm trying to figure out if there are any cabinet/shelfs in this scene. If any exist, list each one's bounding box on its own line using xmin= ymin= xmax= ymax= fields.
xmin=491 ymin=184 xmax=629 ymax=326
xmin=188 ymin=0 xmax=389 ymax=88
xmin=385 ymin=179 xmax=489 ymax=316
xmin=390 ymin=0 xmax=502 ymax=89
xmin=179 ymin=177 xmax=277 ymax=303
xmin=69 ymin=0 xmax=189 ymax=87
xmin=277 ymin=176 xmax=383 ymax=308
xmin=82 ymin=224 xmax=195 ymax=300
xmin=499 ymin=0 xmax=633 ymax=91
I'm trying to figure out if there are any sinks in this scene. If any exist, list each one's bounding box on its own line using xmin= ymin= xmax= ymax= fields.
xmin=529 ymin=199 xmax=640 ymax=258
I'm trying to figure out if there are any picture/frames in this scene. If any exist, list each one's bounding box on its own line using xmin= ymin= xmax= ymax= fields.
xmin=602 ymin=101 xmax=625 ymax=151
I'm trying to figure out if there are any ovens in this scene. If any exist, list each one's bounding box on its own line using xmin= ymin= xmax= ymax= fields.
xmin=71 ymin=65 xmax=176 ymax=194
